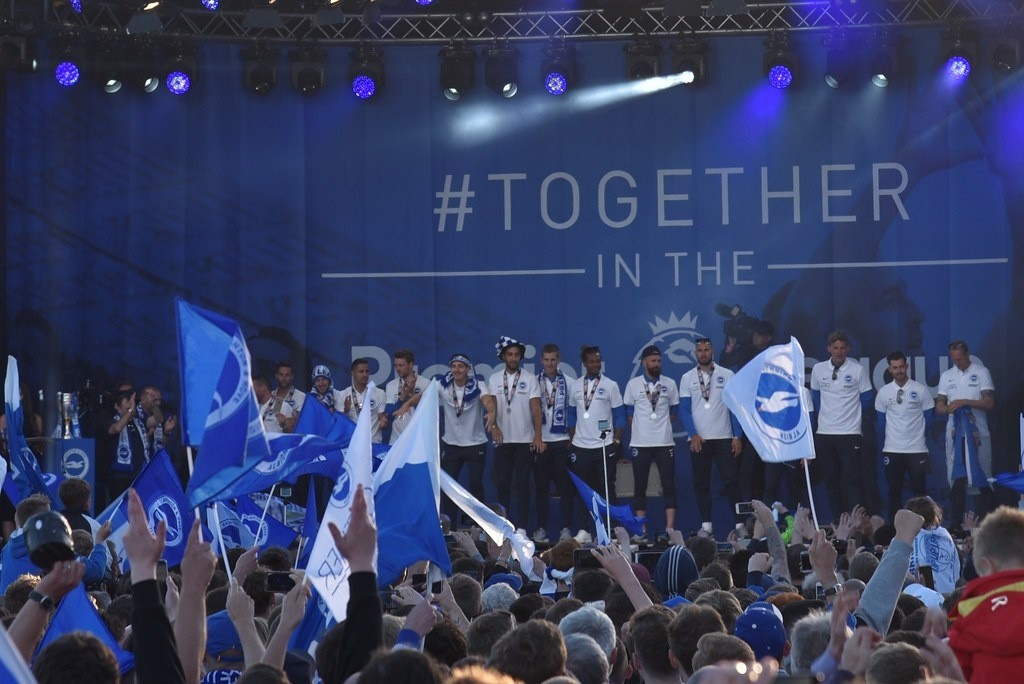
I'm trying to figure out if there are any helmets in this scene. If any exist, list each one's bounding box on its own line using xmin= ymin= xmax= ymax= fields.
xmin=23 ymin=510 xmax=75 ymax=568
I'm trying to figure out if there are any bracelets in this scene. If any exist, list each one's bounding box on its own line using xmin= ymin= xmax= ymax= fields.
xmin=162 ymin=429 xmax=171 ymax=436
xmin=127 ymin=409 xmax=134 ymax=415
xmin=497 ymin=556 xmax=508 ymax=564
xmin=848 ymin=536 xmax=856 ymax=539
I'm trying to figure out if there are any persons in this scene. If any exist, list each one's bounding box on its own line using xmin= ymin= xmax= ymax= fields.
xmin=0 ymin=488 xmax=1024 ymax=684
xmin=0 ymin=335 xmax=994 ymax=541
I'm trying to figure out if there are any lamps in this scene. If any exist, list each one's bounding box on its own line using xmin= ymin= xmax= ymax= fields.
xmin=869 ymin=43 xmax=903 ymax=88
xmin=764 ymin=47 xmax=800 ymax=88
xmin=162 ymin=42 xmax=201 ymax=93
xmin=291 ymin=46 xmax=327 ymax=94
xmin=440 ymin=49 xmax=474 ymax=101
xmin=97 ymin=54 xmax=123 ymax=94
xmin=940 ymin=30 xmax=982 ymax=79
xmin=345 ymin=49 xmax=385 ymax=101
xmin=823 ymin=50 xmax=853 ymax=89
xmin=54 ymin=41 xmax=89 ymax=87
xmin=241 ymin=42 xmax=281 ymax=93
xmin=621 ymin=43 xmax=663 ymax=84
xmin=28 ymin=45 xmax=61 ymax=77
xmin=137 ymin=66 xmax=158 ymax=93
xmin=540 ymin=46 xmax=576 ymax=95
xmin=989 ymin=33 xmax=1019 ymax=72
xmin=673 ymin=40 xmax=710 ymax=85
xmin=480 ymin=44 xmax=520 ymax=97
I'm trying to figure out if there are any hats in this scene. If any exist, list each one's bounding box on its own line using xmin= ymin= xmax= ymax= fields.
xmin=312 ymin=365 xmax=331 ymax=387
xmin=734 ymin=602 xmax=787 ymax=659
xmin=641 ymin=345 xmax=660 ymax=356
xmin=495 ymin=336 xmax=525 ymax=362
xmin=825 ymin=603 xmax=856 ymax=632
xmin=484 ymin=573 xmax=522 ymax=592
xmin=663 ymin=596 xmax=692 ymax=608
xmin=207 ymin=610 xmax=244 ymax=662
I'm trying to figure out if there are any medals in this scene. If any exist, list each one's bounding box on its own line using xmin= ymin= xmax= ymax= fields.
xmin=398 ymin=415 xmax=405 ymax=420
xmin=583 ymin=413 xmax=589 ymax=419
xmin=650 ymin=414 xmax=657 ymax=419
xmin=506 ymin=406 xmax=512 ymax=414
xmin=358 ymin=403 xmax=362 ymax=409
xmin=705 ymin=404 xmax=710 ymax=409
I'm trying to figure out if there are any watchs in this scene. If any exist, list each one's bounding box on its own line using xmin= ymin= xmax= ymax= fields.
xmin=823 ymin=583 xmax=843 ymax=596
xmin=27 ymin=591 xmax=54 ymax=612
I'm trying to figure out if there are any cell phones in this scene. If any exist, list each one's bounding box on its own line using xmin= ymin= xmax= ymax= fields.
xmin=157 ymin=560 xmax=169 ymax=585
xmin=573 ymin=548 xmax=604 ymax=570
xmin=412 ymin=574 xmax=427 ymax=593
xmin=432 ymin=580 xmax=443 ymax=595
xmin=735 ymin=502 xmax=756 ymax=514
xmin=919 ymin=566 xmax=934 ymax=591
xmin=379 ymin=589 xmax=399 ymax=604
xmin=800 ymin=551 xmax=813 ymax=573
xmin=263 ymin=572 xmax=296 ymax=593
xmin=444 ymin=535 xmax=455 ymax=544
xmin=816 ymin=582 xmax=825 ymax=601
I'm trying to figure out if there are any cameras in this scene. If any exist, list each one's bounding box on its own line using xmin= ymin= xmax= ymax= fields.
xmin=655 ymin=532 xmax=670 ymax=543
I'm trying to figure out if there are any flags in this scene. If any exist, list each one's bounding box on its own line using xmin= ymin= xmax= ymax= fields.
xmin=722 ymin=336 xmax=816 ymax=461
xmin=0 ymin=298 xmax=654 ymax=674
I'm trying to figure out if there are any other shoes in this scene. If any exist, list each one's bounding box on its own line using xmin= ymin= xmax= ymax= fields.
xmin=574 ymin=530 xmax=592 ymax=543
xmin=559 ymin=528 xmax=571 ymax=542
xmin=531 ymin=528 xmax=549 ymax=542
xmin=735 ymin=525 xmax=749 ymax=540
xmin=698 ymin=528 xmax=713 ymax=539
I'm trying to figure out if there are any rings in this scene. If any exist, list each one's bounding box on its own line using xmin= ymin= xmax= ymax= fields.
xmin=63 ymin=565 xmax=71 ymax=569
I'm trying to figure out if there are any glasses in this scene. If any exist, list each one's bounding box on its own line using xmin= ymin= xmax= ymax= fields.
xmin=948 ymin=342 xmax=964 ymax=350
xmin=695 ymin=338 xmax=711 ymax=345
xmin=897 ymin=389 xmax=903 ymax=403
xmin=832 ymin=366 xmax=838 ymax=379
